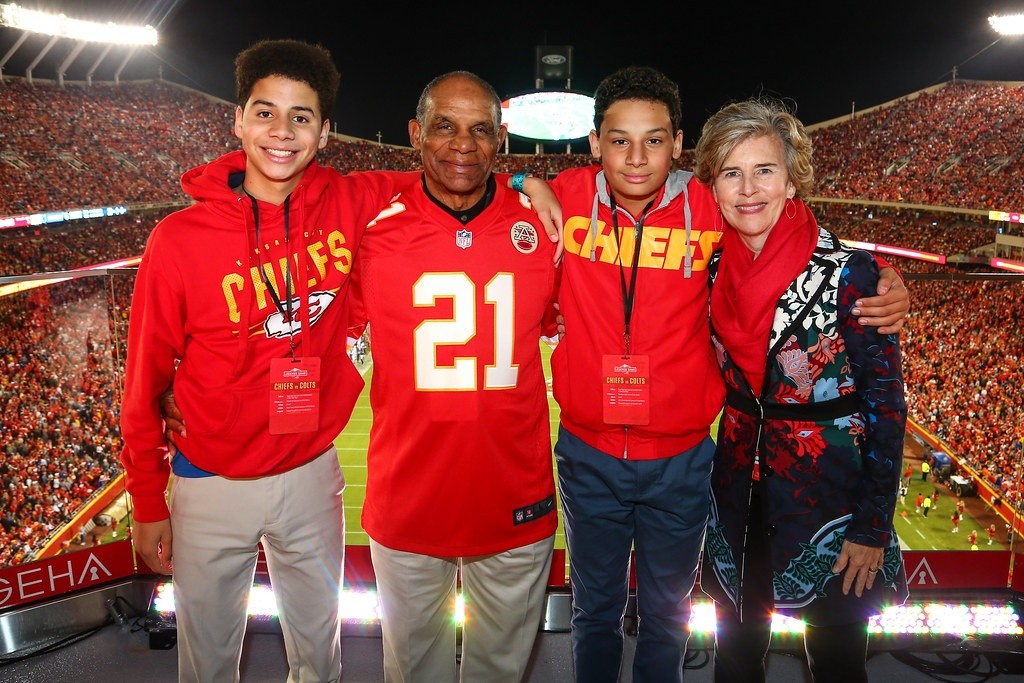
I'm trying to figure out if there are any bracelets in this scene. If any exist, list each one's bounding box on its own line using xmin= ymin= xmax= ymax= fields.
xmin=512 ymin=171 xmax=533 ymax=194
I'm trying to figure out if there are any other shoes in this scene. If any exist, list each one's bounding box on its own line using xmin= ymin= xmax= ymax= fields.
xmin=959 ymin=514 xmax=963 ymax=520
xmin=952 ymin=527 xmax=958 ymax=533
xmin=916 ymin=509 xmax=920 ymax=513
xmin=987 ymin=540 xmax=992 ymax=546
xmin=932 ymin=505 xmax=937 ymax=509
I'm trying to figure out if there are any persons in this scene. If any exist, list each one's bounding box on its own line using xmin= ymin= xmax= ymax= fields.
xmin=346 ymin=328 xmax=372 ymax=364
xmin=803 ymin=82 xmax=1024 ymax=550
xmin=490 ymin=152 xmax=602 ymax=182
xmin=0 ymin=278 xmax=136 ymax=570
xmin=543 ymin=67 xmax=910 ymax=683
xmin=553 ymin=100 xmax=910 ymax=683
xmin=119 ymin=40 xmax=564 ymax=683
xmin=316 ymin=135 xmax=424 ymax=175
xmin=346 ymin=71 xmax=559 ymax=683
xmin=672 ymin=150 xmax=697 ymax=172
xmin=0 ymin=77 xmax=244 ymax=568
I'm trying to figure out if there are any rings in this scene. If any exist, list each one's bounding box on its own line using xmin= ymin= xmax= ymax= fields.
xmin=869 ymin=570 xmax=878 ymax=574
